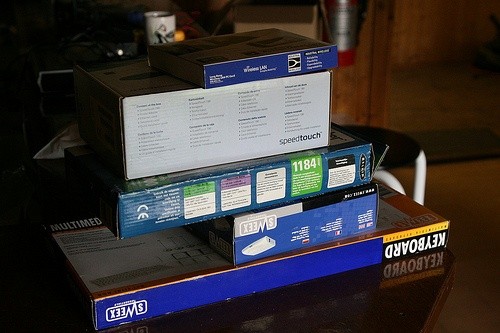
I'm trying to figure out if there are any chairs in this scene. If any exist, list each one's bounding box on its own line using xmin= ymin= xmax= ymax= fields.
xmin=341 ymin=126 xmax=427 ymax=206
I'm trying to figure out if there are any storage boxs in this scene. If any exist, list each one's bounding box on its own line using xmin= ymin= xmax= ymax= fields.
xmin=213 ymin=0 xmax=333 ymax=42
xmin=40 ymin=28 xmax=451 ymax=330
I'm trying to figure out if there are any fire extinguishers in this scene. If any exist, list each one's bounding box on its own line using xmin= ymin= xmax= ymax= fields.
xmin=320 ymin=0 xmax=365 ymax=67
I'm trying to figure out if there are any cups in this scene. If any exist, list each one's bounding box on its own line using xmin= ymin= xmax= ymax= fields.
xmin=144 ymin=10 xmax=176 ymax=43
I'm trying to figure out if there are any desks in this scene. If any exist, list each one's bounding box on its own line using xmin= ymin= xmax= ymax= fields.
xmin=0 ymin=210 xmax=457 ymax=333
